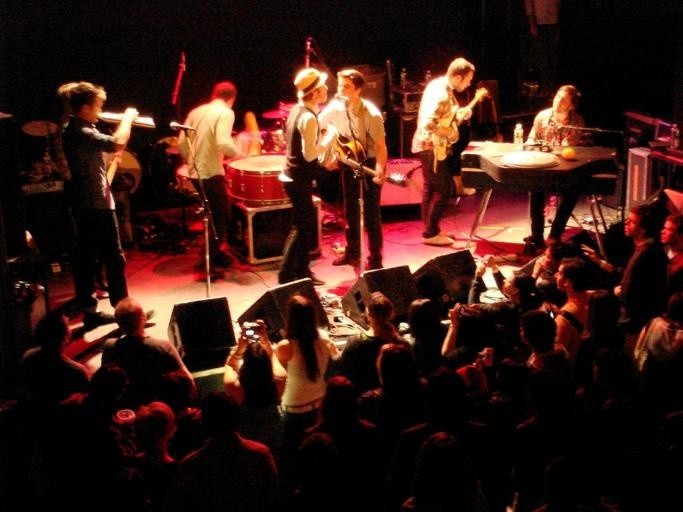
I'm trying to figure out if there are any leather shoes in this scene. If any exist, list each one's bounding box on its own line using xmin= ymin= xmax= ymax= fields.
xmin=423 ymin=233 xmax=454 ymax=246
xmin=87 ymin=313 xmax=116 ymax=325
xmin=333 ymin=254 xmax=360 ymax=265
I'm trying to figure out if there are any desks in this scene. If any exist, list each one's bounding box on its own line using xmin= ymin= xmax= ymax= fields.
xmin=460 ymin=140 xmax=617 ymax=249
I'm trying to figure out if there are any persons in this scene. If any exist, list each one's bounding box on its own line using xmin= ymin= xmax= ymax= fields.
xmin=524 ymin=84 xmax=586 ymax=246
xmin=278 ymin=67 xmax=338 ymax=287
xmin=411 ymin=57 xmax=476 ymax=246
xmin=58 ymin=80 xmax=155 ymax=328
xmin=1 ymin=189 xmax=682 ymax=512
xmin=179 ymin=80 xmax=242 ymax=268
xmin=446 ymin=121 xmax=476 ymax=195
xmin=319 ymin=69 xmax=388 ymax=271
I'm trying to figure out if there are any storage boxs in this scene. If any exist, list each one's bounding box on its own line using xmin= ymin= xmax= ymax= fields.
xmin=229 ymin=195 xmax=322 ymax=264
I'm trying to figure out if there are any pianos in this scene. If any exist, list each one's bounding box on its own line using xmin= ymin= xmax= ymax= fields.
xmin=460 ymin=140 xmax=619 ymax=196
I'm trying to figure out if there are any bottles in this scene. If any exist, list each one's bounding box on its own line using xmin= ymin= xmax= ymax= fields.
xmin=426 ymin=71 xmax=432 ymax=82
xmin=401 ymin=68 xmax=408 ymax=90
xmin=513 ymin=123 xmax=524 ymax=151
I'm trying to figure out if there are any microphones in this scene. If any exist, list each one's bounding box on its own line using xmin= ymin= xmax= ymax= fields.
xmin=557 ymin=122 xmax=581 ymax=134
xmin=332 ymin=93 xmax=348 ymax=103
xmin=169 ymin=118 xmax=196 ymax=132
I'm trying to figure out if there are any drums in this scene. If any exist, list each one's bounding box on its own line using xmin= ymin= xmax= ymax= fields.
xmin=225 ymin=154 xmax=293 ymax=207
xmin=175 ymin=163 xmax=210 ymax=197
xmin=166 ymin=147 xmax=182 ymax=165
xmin=116 ymin=148 xmax=143 ymax=194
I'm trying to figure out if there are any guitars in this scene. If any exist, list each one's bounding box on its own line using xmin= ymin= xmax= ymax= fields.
xmin=316 ymin=129 xmax=407 ymax=187
xmin=432 ymin=88 xmax=488 ymax=162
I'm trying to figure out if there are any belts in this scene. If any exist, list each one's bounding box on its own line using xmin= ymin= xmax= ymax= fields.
xmin=282 ymin=401 xmax=322 ymax=413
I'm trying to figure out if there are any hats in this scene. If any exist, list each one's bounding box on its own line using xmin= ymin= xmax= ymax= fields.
xmin=294 ymin=68 xmax=328 ymax=99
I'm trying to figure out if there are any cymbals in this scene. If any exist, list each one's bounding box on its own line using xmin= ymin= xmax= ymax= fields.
xmin=22 ymin=121 xmax=58 ymax=137
xmin=262 ymin=110 xmax=289 ymax=119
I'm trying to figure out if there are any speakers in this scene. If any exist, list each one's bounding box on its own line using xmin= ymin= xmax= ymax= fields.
xmin=412 ymin=249 xmax=487 ymax=304
xmin=340 ymin=264 xmax=423 ymax=331
xmin=166 ymin=295 xmax=236 ymax=370
xmin=238 ymin=278 xmax=331 ymax=341
